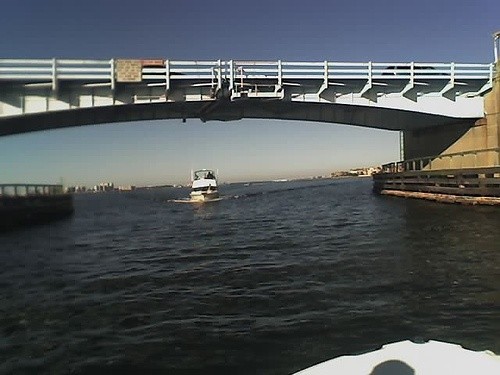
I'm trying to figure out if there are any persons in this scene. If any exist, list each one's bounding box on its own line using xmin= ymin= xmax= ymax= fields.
xmin=206 ymin=170 xmax=214 ymax=179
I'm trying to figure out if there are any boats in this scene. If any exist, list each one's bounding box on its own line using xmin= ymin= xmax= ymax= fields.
xmin=189 ymin=167 xmax=218 ymax=202
